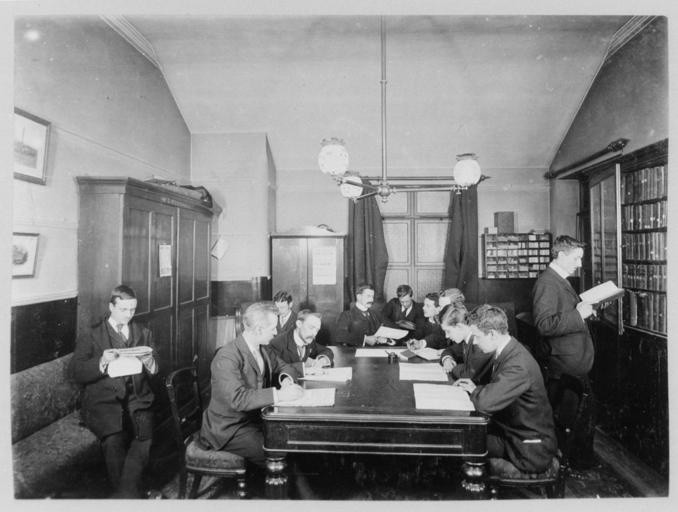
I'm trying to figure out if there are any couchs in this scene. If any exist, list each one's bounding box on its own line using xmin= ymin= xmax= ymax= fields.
xmin=10 ymin=350 xmax=103 ymax=499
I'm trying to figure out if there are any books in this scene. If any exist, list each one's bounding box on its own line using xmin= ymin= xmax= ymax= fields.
xmin=579 ymin=165 xmax=668 ymax=335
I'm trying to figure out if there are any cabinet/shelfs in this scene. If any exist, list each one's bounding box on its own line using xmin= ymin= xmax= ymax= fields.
xmin=271 ymin=234 xmax=354 ymax=322
xmin=588 ymin=164 xmax=667 ymax=342
xmin=77 ymin=174 xmax=218 ymax=431
xmin=479 ymin=231 xmax=554 ymax=280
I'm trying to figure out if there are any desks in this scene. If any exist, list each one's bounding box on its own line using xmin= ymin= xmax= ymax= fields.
xmin=260 ymin=346 xmax=491 ymax=499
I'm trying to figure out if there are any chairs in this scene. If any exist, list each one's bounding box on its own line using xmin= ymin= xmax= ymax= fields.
xmin=491 ymin=373 xmax=583 ymax=499
xmin=166 ymin=365 xmax=249 ymax=499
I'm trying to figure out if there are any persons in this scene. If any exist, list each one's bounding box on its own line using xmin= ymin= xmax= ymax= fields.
xmin=452 ymin=303 xmax=560 ymax=474
xmin=531 ymin=236 xmax=613 ymax=469
xmin=384 ymin=284 xmax=465 ymax=350
xmin=334 ymin=285 xmax=396 ymax=348
xmin=440 ymin=303 xmax=493 ymax=381
xmin=273 ymin=290 xmax=297 ymax=333
xmin=72 ymin=285 xmax=160 ymax=499
xmin=268 ymin=308 xmax=334 ymax=377
xmin=198 ymin=301 xmax=318 ymax=500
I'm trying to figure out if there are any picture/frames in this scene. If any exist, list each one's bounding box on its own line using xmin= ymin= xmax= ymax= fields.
xmin=13 ymin=232 xmax=40 ymax=278
xmin=13 ymin=107 xmax=52 ymax=186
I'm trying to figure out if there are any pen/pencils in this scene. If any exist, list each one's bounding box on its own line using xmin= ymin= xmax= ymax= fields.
xmin=410 ymin=338 xmax=412 ymax=351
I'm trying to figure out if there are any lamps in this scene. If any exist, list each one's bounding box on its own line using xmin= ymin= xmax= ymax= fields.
xmin=318 ymin=16 xmax=491 ymax=202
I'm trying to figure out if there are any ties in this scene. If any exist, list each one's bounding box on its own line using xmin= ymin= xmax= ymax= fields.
xmin=117 ymin=324 xmax=127 ymax=343
xmin=363 ymin=310 xmax=370 ymax=318
xmin=403 ymin=308 xmax=407 ymax=315
